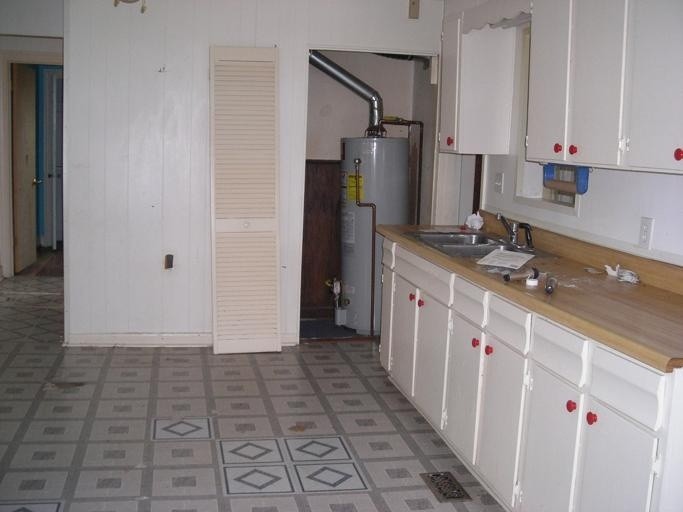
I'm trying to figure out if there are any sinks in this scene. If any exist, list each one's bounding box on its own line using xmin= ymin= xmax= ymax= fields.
xmin=434 ymin=243 xmax=528 ymax=258
xmin=401 ymin=230 xmax=501 ymax=245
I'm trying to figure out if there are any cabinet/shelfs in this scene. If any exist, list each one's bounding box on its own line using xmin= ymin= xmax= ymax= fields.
xmin=615 ymin=1 xmax=682 ymax=173
xmin=523 ymin=3 xmax=626 ymax=166
xmin=437 ymin=11 xmax=513 ymax=156
xmin=520 ymin=316 xmax=665 ymax=512
xmin=444 ymin=276 xmax=531 ymax=509
xmin=391 ymin=244 xmax=454 ymax=426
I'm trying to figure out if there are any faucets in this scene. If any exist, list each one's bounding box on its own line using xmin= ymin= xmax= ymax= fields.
xmin=495 ymin=211 xmax=520 ymax=246
